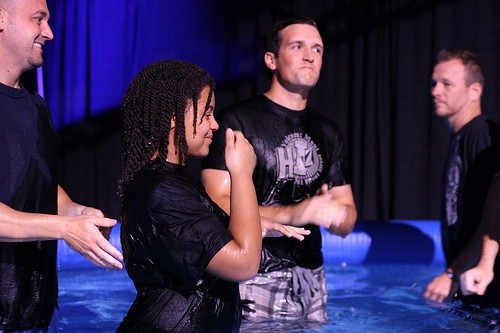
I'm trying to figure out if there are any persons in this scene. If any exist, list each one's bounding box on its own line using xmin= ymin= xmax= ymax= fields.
xmin=423 ymin=43 xmax=500 ymax=306
xmin=115 ymin=59 xmax=312 ymax=333
xmin=200 ymin=17 xmax=358 ymax=333
xmin=0 ymin=0 xmax=124 ymax=333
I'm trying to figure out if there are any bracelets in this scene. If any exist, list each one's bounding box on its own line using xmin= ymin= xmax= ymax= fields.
xmin=445 ymin=265 xmax=463 ymax=281
